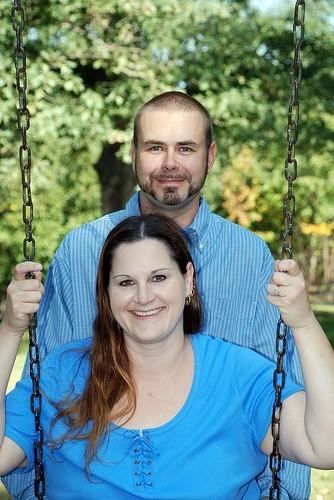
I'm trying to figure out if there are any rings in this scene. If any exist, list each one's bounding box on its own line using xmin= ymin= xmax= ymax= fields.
xmin=273 ymin=284 xmax=281 ymax=295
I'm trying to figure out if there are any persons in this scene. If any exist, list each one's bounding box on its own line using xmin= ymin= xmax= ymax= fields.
xmin=0 ymin=213 xmax=334 ymax=500
xmin=23 ymin=90 xmax=312 ymax=500
